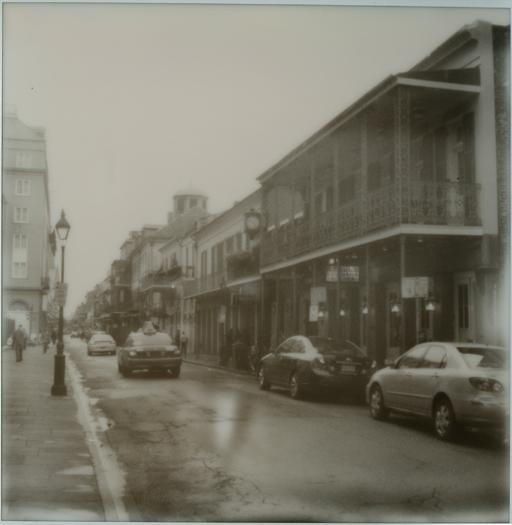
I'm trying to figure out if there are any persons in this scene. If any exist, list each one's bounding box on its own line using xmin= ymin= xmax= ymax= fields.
xmin=13 ymin=324 xmax=25 ymax=362
xmin=179 ymin=330 xmax=189 ymax=361
xmin=41 ymin=326 xmax=57 ymax=355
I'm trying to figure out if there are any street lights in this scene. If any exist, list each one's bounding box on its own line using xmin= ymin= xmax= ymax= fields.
xmin=48 ymin=209 xmax=72 ymax=397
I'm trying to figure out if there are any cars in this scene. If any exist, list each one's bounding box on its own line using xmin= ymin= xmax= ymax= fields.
xmin=253 ymin=334 xmax=377 ymax=401
xmin=365 ymin=342 xmax=511 ymax=445
xmin=116 ymin=321 xmax=182 ymax=379
xmin=68 ymin=328 xmax=116 ymax=356
